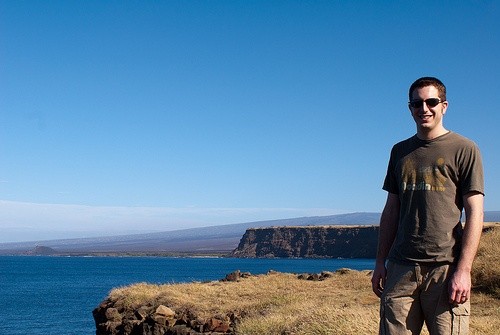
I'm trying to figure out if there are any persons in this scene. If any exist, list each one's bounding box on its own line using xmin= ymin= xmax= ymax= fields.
xmin=372 ymin=77 xmax=485 ymax=335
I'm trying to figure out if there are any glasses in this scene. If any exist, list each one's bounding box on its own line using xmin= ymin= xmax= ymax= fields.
xmin=407 ymin=99 xmax=445 ymax=107
